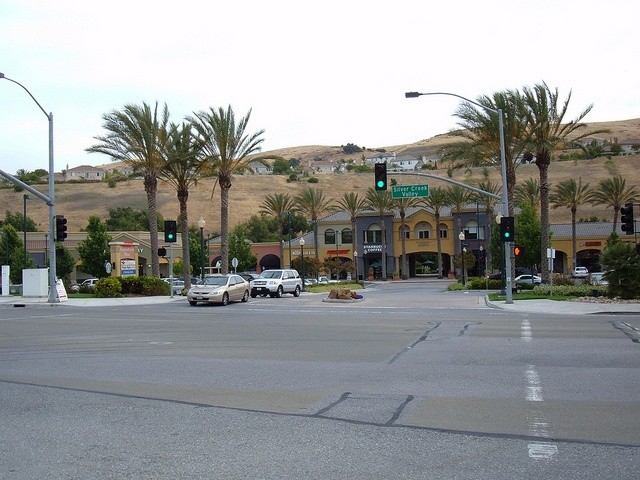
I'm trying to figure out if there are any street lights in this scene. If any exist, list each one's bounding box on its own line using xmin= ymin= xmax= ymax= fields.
xmin=364 ymin=250 xmax=368 ymax=280
xmin=405 ymin=92 xmax=513 ymax=303
xmin=458 ymin=232 xmax=467 ymax=286
xmin=479 ymin=246 xmax=483 ymax=278
xmin=198 ymin=217 xmax=205 ymax=279
xmin=299 ymin=237 xmax=305 ymax=289
xmin=496 ymin=212 xmax=505 ymax=294
xmin=24 ymin=194 xmax=30 ymax=268
xmin=354 ymin=251 xmax=358 ymax=283
xmin=0 ymin=71 xmax=62 ymax=301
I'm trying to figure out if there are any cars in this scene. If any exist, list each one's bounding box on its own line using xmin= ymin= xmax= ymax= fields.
xmin=318 ymin=275 xmax=329 ymax=284
xmin=240 ymin=273 xmax=259 ymax=281
xmin=571 ymin=267 xmax=589 ymax=278
xmin=187 ymin=273 xmax=251 ymax=305
xmin=590 ymin=272 xmax=609 ymax=285
xmin=173 ymin=280 xmax=185 ymax=292
xmin=304 ymin=279 xmax=317 ymax=285
xmin=487 ymin=267 xmax=538 ymax=279
xmin=515 ymin=275 xmax=541 ymax=284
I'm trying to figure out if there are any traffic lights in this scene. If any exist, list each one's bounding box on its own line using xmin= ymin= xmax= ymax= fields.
xmin=514 ymin=247 xmax=521 ymax=256
xmin=501 ymin=217 xmax=514 ymax=241
xmin=164 ymin=220 xmax=176 ymax=242
xmin=621 ymin=203 xmax=634 ymax=235
xmin=375 ymin=163 xmax=387 ymax=190
xmin=56 ymin=214 xmax=68 ymax=241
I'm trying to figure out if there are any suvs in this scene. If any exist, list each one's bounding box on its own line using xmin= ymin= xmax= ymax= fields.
xmin=70 ymin=279 xmax=99 ymax=292
xmin=250 ymin=269 xmax=302 ymax=298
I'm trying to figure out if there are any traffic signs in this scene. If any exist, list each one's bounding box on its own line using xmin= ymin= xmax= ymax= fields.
xmin=392 ymin=184 xmax=429 ymax=199
